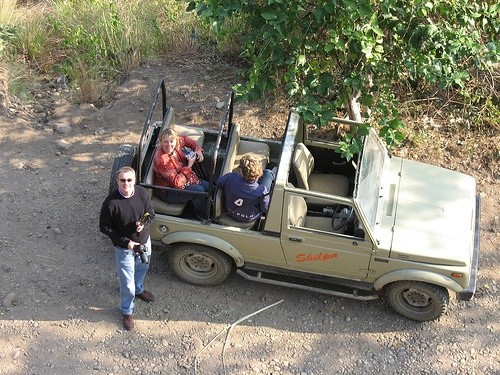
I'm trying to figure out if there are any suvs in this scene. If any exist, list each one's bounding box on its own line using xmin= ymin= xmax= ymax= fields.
xmin=107 ymin=76 xmax=482 ymax=323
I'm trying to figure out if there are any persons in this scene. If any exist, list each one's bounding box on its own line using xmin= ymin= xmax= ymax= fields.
xmin=152 ymin=128 xmax=216 ymax=223
xmin=99 ymin=166 xmax=156 ymax=331
xmin=216 ymin=152 xmax=278 ymax=221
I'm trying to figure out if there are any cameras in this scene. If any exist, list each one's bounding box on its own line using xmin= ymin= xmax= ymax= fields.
xmin=185 ymin=150 xmax=196 ymax=160
xmin=133 ymin=245 xmax=149 ymax=265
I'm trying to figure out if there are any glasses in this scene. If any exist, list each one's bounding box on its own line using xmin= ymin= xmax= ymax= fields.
xmin=119 ymin=178 xmax=133 ymax=182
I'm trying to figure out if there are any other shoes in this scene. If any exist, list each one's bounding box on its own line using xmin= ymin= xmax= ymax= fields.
xmin=137 ymin=289 xmax=155 ymax=302
xmin=123 ymin=315 xmax=134 ymax=330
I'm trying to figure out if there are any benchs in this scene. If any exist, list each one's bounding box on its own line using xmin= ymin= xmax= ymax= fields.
xmin=145 ymin=107 xmax=204 ymax=216
xmin=216 ymin=122 xmax=271 ymax=230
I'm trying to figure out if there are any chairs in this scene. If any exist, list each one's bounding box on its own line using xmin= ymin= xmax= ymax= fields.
xmin=288 ymin=183 xmax=347 ymax=235
xmin=292 ymin=143 xmax=349 ymax=199
